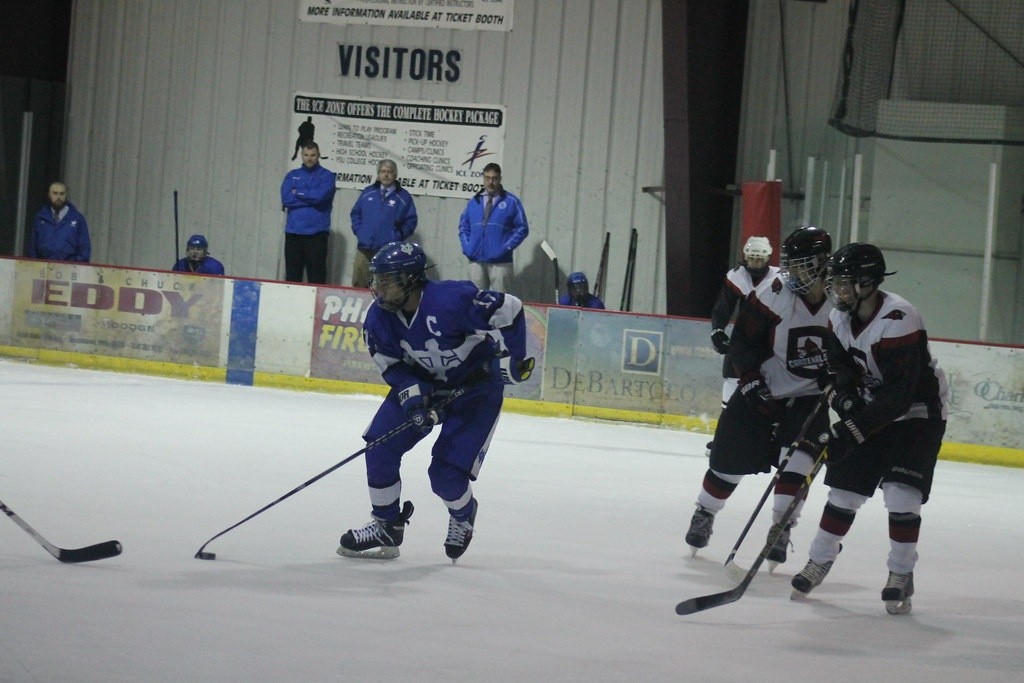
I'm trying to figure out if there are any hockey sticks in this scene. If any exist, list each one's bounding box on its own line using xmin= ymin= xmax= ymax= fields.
xmin=195 ymin=388 xmax=465 ymax=559
xmin=724 ymin=386 xmax=759 ymax=587
xmin=675 ymin=445 xmax=832 ymax=615
xmin=0 ymin=501 xmax=122 ymax=563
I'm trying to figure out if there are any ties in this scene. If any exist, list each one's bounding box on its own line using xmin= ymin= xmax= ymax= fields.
xmin=484 ymin=194 xmax=493 ymax=224
xmin=51 ymin=207 xmax=61 ymax=224
xmin=380 ymin=188 xmax=389 ymax=201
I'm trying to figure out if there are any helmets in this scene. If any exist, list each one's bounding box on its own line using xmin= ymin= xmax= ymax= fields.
xmin=821 ymin=243 xmax=888 ymax=317
xmin=565 ymin=271 xmax=586 ymax=287
xmin=368 ymin=241 xmax=427 ymax=311
xmin=742 ymin=235 xmax=773 ymax=259
xmin=779 ymin=226 xmax=832 ymax=295
xmin=187 ymin=234 xmax=209 ymax=251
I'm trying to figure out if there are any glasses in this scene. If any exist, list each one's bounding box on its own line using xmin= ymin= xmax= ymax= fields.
xmin=482 ymin=174 xmax=501 ymax=182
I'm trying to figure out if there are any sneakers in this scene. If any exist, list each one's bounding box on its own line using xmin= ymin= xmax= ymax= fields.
xmin=765 ymin=521 xmax=795 ymax=573
xmin=880 ymin=572 xmax=916 ymax=615
xmin=685 ymin=505 xmax=715 ymax=558
xmin=444 ymin=494 xmax=479 ymax=565
xmin=705 ymin=441 xmax=714 ymax=457
xmin=789 ymin=543 xmax=843 ymax=599
xmin=336 ymin=499 xmax=415 ymax=560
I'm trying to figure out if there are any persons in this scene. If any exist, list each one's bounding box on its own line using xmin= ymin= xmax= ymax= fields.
xmin=280 ymin=142 xmax=335 ymax=283
xmin=336 ymin=241 xmax=536 ymax=566
xmin=349 ymin=158 xmax=418 ymax=289
xmin=559 ymin=272 xmax=605 ymax=309
xmin=28 ymin=180 xmax=91 ymax=264
xmin=790 ymin=242 xmax=948 ymax=615
xmin=458 ymin=163 xmax=529 ymax=295
xmin=705 ymin=235 xmax=781 ymax=459
xmin=171 ymin=234 xmax=224 ymax=275
xmin=685 ymin=227 xmax=838 ymax=574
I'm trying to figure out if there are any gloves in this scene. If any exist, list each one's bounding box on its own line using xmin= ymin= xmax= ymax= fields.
xmin=816 ymin=360 xmax=836 ymax=392
xmin=823 ymin=382 xmax=867 ymax=421
xmin=738 ymin=373 xmax=786 ymax=421
xmin=493 ymin=353 xmax=535 ymax=385
xmin=398 ymin=379 xmax=434 ymax=429
xmin=711 ymin=329 xmax=732 ymax=355
xmin=812 ymin=418 xmax=870 ymax=466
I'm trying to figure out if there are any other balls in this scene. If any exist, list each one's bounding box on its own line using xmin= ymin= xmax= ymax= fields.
xmin=199 ymin=552 xmax=216 ymax=560
